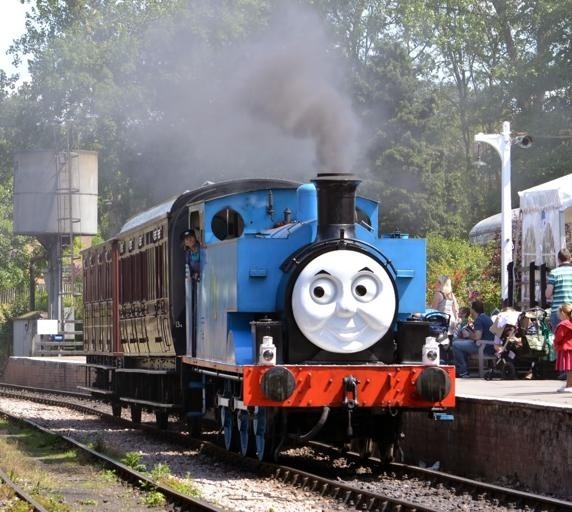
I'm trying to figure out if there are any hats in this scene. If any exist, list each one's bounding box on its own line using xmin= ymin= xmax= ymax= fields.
xmin=180 ymin=230 xmax=194 ymax=240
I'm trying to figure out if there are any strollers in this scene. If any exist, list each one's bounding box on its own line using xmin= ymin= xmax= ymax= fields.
xmin=420 ymin=309 xmax=451 ymax=366
xmin=484 ymin=306 xmax=553 ymax=380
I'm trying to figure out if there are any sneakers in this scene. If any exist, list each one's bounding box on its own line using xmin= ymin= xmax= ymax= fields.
xmin=459 ymin=373 xmax=471 ymax=377
xmin=557 ymin=387 xmax=572 ymax=392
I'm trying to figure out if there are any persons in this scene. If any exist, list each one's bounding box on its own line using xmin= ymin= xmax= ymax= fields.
xmin=431 ymin=249 xmax=572 ymax=392
xmin=179 ymin=228 xmax=201 ymax=280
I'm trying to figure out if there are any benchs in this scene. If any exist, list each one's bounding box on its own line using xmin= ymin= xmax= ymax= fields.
xmin=470 ymin=338 xmax=506 ymax=378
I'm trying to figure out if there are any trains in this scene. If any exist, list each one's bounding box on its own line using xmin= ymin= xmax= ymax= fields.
xmin=78 ymin=171 xmax=457 ymax=465
